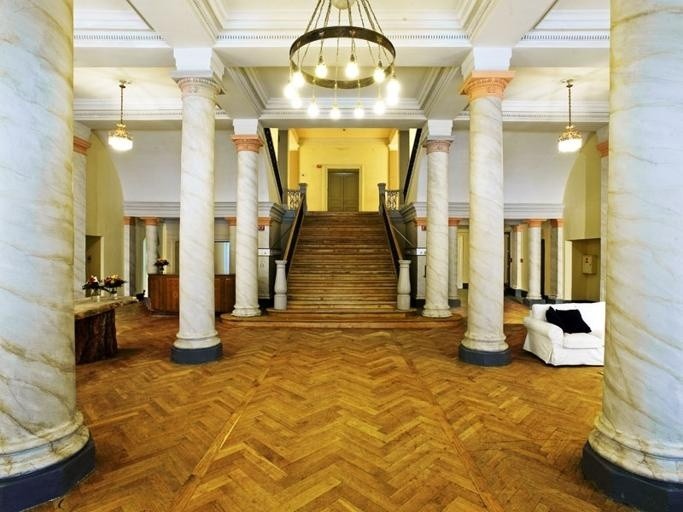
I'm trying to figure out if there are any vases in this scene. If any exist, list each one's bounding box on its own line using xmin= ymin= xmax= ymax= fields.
xmin=158 ymin=266 xmax=164 ymax=272
xmin=90 ymin=288 xmax=117 ymax=302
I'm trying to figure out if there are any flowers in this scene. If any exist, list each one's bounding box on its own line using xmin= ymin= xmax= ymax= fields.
xmin=81 ymin=272 xmax=124 ymax=296
xmin=153 ymin=258 xmax=168 ymax=266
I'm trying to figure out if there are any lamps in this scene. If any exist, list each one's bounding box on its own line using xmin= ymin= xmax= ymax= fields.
xmin=280 ymin=1 xmax=404 ymax=122
xmin=105 ymin=79 xmax=134 ymax=152
xmin=556 ymin=77 xmax=582 ymax=154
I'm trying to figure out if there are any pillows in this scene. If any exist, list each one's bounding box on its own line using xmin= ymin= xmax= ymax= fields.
xmin=544 ymin=306 xmax=594 ymax=335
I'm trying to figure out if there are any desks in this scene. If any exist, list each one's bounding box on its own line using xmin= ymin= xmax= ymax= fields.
xmin=147 ymin=272 xmax=234 ymax=316
xmin=74 ymin=295 xmax=137 ymax=366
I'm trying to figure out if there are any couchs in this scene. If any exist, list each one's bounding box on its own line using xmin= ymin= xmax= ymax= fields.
xmin=520 ymin=300 xmax=605 ymax=369
